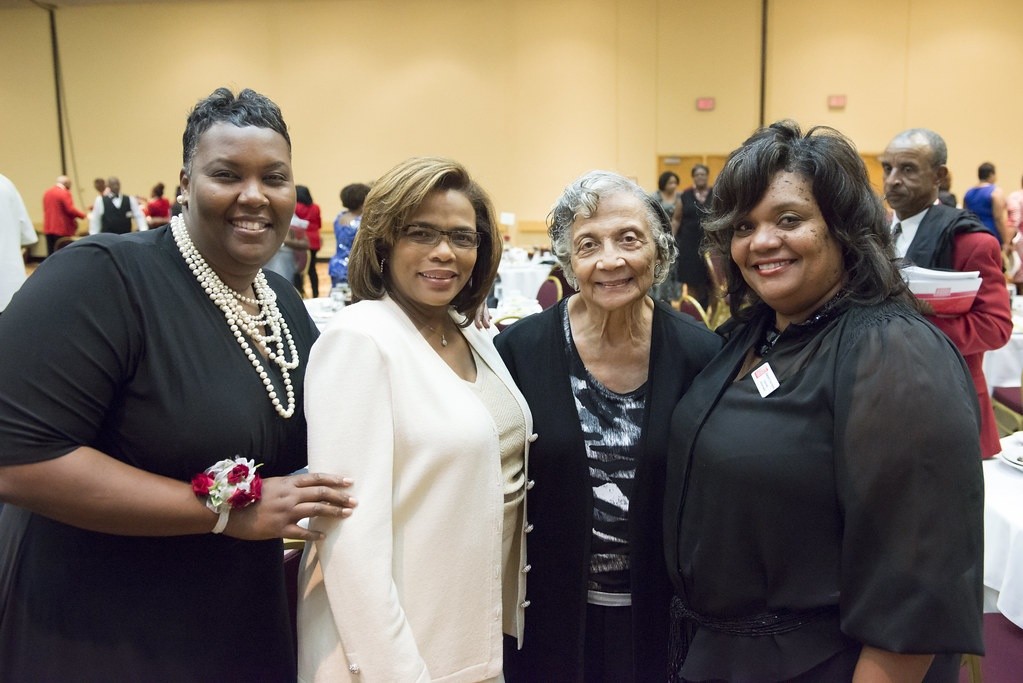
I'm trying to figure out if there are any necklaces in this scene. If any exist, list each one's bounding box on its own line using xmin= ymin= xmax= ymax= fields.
xmin=392 ymin=297 xmax=447 ymax=346
xmin=169 ymin=213 xmax=299 ymax=418
xmin=754 ymin=284 xmax=847 ymax=359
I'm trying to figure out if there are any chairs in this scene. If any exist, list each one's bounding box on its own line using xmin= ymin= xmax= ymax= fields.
xmin=703 ymin=246 xmax=748 ymax=328
xmin=494 ymin=276 xmax=563 ymax=331
xmin=679 ymin=295 xmax=711 ymax=330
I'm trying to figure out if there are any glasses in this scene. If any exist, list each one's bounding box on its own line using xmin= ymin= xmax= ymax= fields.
xmin=389 ymin=222 xmax=487 ymax=250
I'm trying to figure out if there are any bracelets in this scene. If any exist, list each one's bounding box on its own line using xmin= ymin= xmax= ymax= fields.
xmin=192 ymin=454 xmax=262 ymax=533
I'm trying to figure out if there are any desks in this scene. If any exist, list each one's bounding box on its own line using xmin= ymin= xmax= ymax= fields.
xmin=982 ymin=294 xmax=1023 ymax=392
xmin=980 ymin=430 xmax=1023 ymax=631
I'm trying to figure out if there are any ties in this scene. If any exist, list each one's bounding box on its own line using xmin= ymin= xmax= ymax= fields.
xmin=889 ymin=222 xmax=903 ymax=243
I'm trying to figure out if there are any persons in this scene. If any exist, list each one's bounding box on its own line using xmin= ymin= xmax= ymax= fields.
xmin=0 ymin=175 xmax=39 ymax=312
xmin=329 ymin=184 xmax=371 ymax=286
xmin=938 ymin=173 xmax=957 ymax=208
xmin=0 ymin=85 xmax=494 ymax=683
xmin=881 ymin=128 xmax=1014 ymax=458
xmin=963 ymin=163 xmax=1008 ymax=249
xmin=295 ymin=157 xmax=539 ymax=683
xmin=294 ymin=185 xmax=324 ymax=298
xmin=44 ymin=176 xmax=86 ymax=256
xmin=1007 ymin=175 xmax=1023 ymax=295
xmin=651 ymin=164 xmax=714 ymax=313
xmin=493 ymin=169 xmax=724 ymax=683
xmin=89 ymin=175 xmax=181 ymax=235
xmin=662 ymin=125 xmax=986 ymax=683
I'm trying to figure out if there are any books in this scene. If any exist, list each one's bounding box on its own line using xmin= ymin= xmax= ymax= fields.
xmin=898 ymin=266 xmax=983 ymax=317
